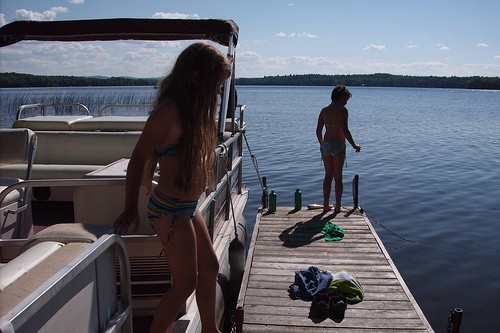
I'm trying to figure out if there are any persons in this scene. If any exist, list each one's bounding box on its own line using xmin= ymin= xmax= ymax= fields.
xmin=314 ymin=85 xmax=363 ymax=214
xmin=110 ymin=42 xmax=233 ymax=333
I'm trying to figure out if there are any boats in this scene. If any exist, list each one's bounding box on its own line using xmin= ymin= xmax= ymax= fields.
xmin=0 ymin=20 xmax=250 ymax=333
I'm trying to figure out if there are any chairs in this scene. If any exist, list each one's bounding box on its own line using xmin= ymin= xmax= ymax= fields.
xmin=0 ymin=128 xmax=35 ymax=240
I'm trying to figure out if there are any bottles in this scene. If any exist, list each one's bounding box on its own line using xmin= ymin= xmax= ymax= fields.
xmin=268 ymin=190 xmax=276 ymax=212
xmin=294 ymin=188 xmax=302 ymax=210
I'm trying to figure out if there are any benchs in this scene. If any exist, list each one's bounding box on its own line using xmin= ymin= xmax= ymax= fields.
xmin=13 ymin=103 xmax=93 ymax=131
xmin=0 ymin=129 xmax=143 ymax=201
xmin=70 ymin=104 xmax=246 ymax=130
